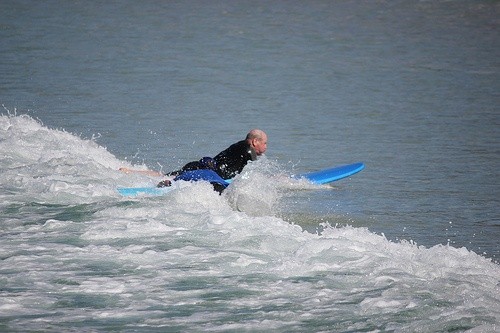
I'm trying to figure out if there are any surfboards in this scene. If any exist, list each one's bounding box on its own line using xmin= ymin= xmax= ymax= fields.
xmin=117 ymin=163 xmax=365 ymax=197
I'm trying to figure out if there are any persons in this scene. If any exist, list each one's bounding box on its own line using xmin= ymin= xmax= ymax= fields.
xmin=120 ymin=128 xmax=267 ymax=193
